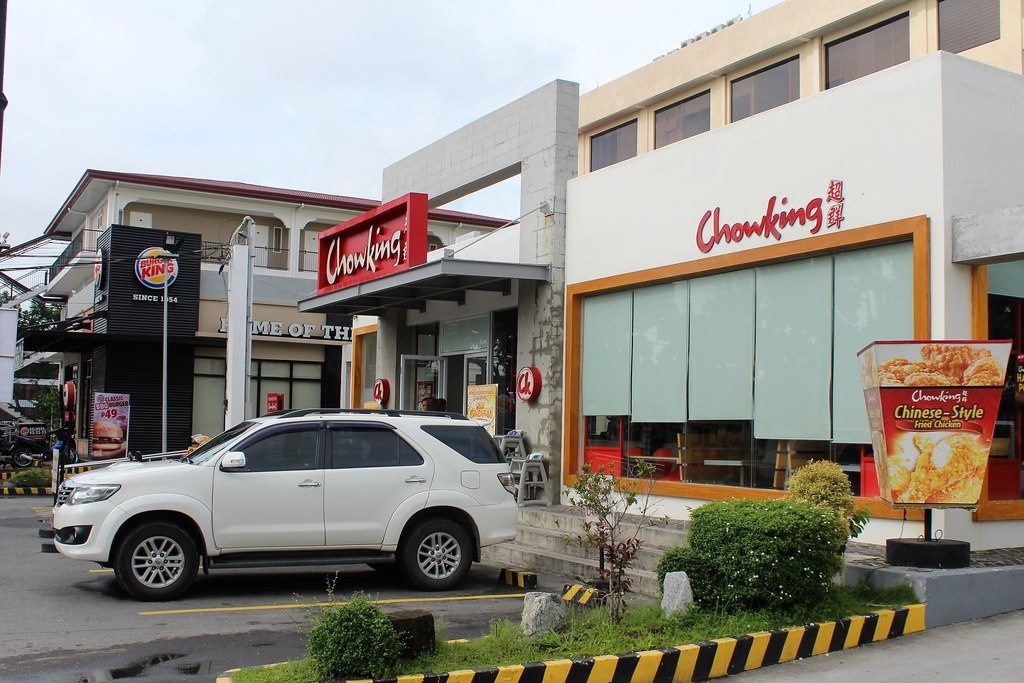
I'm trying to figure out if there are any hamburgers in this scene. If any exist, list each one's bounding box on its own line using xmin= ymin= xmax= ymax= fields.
xmin=92 ymin=421 xmax=123 ymax=450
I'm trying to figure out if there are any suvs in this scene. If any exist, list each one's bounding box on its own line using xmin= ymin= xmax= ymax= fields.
xmin=50 ymin=407 xmax=519 ymax=605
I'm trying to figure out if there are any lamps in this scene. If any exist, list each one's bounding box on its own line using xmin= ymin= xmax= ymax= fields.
xmin=584 ymin=474 xmax=615 ymax=535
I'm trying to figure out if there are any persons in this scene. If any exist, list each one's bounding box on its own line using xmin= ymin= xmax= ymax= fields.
xmin=422 ymin=398 xmax=446 ymax=412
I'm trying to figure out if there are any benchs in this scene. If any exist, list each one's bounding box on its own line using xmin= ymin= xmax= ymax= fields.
xmin=677 ymin=432 xmax=840 ymax=491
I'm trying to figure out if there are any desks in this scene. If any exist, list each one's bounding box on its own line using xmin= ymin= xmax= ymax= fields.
xmin=837 ymin=463 xmax=861 ymax=473
xmin=623 ymin=455 xmax=678 ymax=463
xmin=703 ymin=458 xmax=760 ymax=487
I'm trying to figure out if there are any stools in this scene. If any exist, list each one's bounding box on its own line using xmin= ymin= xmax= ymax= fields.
xmin=493 ymin=435 xmax=554 ymax=506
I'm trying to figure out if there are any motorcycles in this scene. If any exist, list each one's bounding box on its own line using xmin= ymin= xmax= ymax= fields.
xmin=8 ymin=421 xmax=79 ymax=469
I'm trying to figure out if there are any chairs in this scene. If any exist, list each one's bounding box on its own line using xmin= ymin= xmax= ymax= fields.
xmin=623 ymin=447 xmax=679 ymax=482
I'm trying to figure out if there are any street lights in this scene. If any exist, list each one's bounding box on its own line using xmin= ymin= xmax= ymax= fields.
xmin=154 ymin=253 xmax=180 ymax=461
